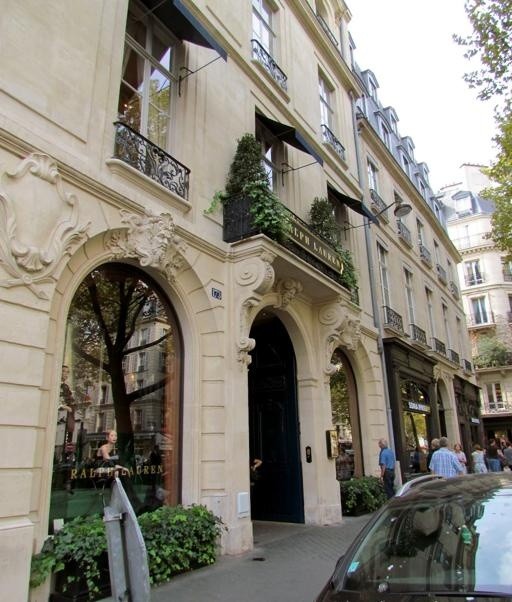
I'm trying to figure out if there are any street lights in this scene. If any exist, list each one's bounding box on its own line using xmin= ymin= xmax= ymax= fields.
xmin=349 ymin=91 xmax=412 ymax=462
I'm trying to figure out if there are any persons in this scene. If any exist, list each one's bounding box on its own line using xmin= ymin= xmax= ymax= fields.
xmin=90 ymin=430 xmax=120 ymax=460
xmin=249 ymin=457 xmax=265 ymax=473
xmin=375 ymin=439 xmax=396 ymax=501
xmin=424 ymin=430 xmax=512 ymax=480
xmin=59 ymin=363 xmax=81 ymax=445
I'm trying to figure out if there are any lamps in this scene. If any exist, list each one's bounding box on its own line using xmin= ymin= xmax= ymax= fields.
xmin=344 ymin=200 xmax=413 ymax=232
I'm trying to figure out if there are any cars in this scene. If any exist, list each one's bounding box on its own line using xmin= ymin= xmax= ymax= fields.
xmin=96 ymin=454 xmax=143 ymax=488
xmin=338 ymin=440 xmax=352 ymax=449
xmin=315 ymin=471 xmax=512 ymax=602
